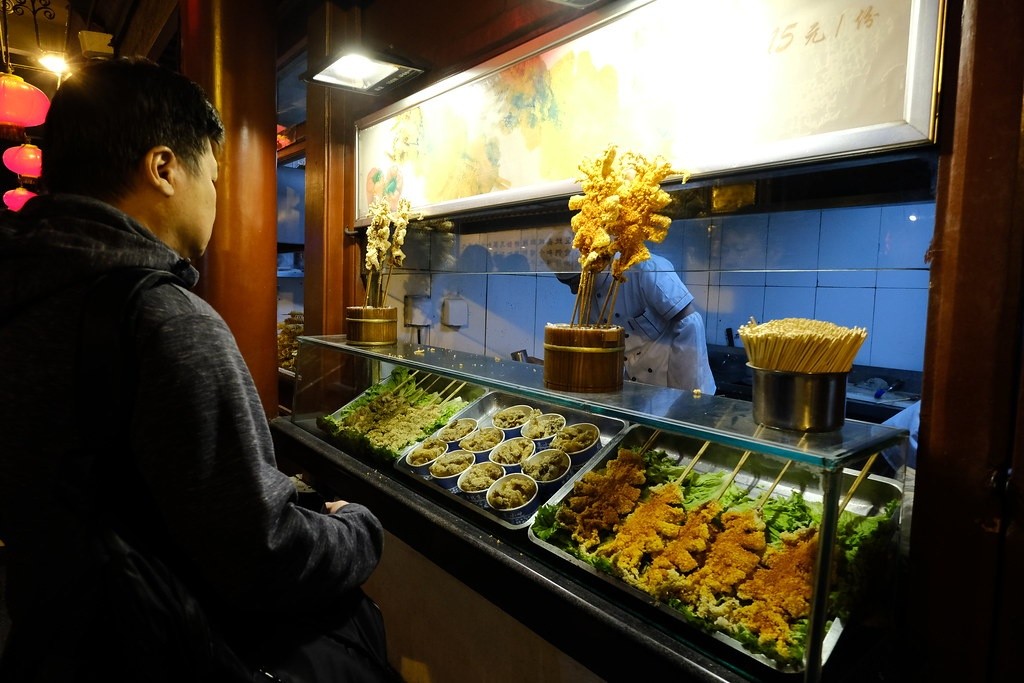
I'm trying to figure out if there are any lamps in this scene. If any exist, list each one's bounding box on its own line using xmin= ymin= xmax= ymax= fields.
xmin=297 ymin=40 xmax=432 ymax=98
xmin=36 ymin=51 xmax=72 ymax=81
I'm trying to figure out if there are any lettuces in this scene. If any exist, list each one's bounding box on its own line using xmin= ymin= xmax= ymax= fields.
xmin=315 ymin=365 xmax=470 ymax=463
xmin=530 ymin=446 xmax=901 ymax=666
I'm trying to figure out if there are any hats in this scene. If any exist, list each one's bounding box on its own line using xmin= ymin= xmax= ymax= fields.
xmin=540 ymin=230 xmax=583 ymax=279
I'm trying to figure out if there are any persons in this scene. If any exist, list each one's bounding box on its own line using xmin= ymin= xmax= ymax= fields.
xmin=539 ymin=227 xmax=717 ymax=395
xmin=878 ymin=398 xmax=922 ymax=476
xmin=0 ymin=56 xmax=382 ymax=683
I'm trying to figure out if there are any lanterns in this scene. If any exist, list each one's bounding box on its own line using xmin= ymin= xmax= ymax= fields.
xmin=0 ymin=72 xmax=50 ymax=139
xmin=3 ymin=188 xmax=38 ymax=213
xmin=2 ymin=138 xmax=43 ymax=185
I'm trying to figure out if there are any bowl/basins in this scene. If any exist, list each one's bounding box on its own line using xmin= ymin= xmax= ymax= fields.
xmin=405 ymin=439 xmax=450 ymax=483
xmin=491 ymin=405 xmax=534 ymax=441
xmin=430 ymin=449 xmax=476 ymax=501
xmin=488 ymin=437 xmax=537 ymax=476
xmin=437 ymin=417 xmax=479 ymax=451
xmin=458 ymin=427 xmax=505 ymax=464
xmin=549 ymin=422 xmax=603 ymax=474
xmin=457 ymin=462 xmax=506 ymax=512
xmin=520 ymin=413 xmax=566 ymax=453
xmin=486 ymin=473 xmax=540 ymax=525
xmin=520 ymin=449 xmax=572 ymax=505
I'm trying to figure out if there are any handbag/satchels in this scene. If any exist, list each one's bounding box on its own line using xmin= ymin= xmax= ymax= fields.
xmin=240 ymin=584 xmax=406 ymax=683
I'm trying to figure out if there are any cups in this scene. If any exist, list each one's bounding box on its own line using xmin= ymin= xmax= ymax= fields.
xmin=511 ymin=350 xmax=528 ymax=363
